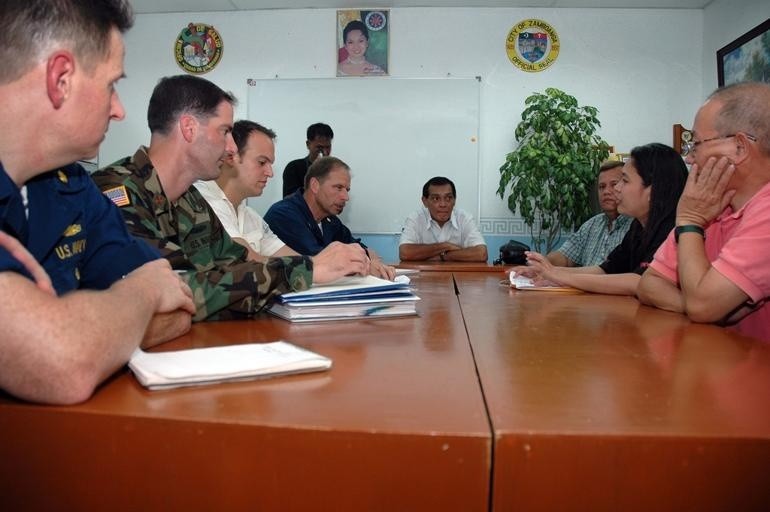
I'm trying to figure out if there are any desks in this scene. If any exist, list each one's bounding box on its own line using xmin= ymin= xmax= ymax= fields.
xmin=0 ymin=259 xmax=770 ymax=511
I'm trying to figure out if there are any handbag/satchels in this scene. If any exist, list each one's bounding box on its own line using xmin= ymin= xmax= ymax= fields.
xmin=499 ymin=240 xmax=528 ymax=264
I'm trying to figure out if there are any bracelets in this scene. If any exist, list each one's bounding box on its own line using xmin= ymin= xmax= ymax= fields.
xmin=441 ymin=252 xmax=445 ymax=262
xmin=674 ymin=225 xmax=704 ymax=242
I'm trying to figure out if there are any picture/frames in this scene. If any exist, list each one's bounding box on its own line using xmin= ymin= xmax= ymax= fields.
xmin=336 ymin=9 xmax=390 ymax=76
xmin=716 ymin=18 xmax=770 ymax=89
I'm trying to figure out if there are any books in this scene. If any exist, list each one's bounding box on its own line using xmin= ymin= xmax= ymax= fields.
xmin=129 ymin=338 xmax=331 ymax=391
xmin=265 ymin=268 xmax=421 ymax=324
xmin=509 ymin=271 xmax=584 ymax=294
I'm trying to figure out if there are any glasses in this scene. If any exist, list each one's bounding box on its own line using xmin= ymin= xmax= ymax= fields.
xmin=682 ymin=133 xmax=756 ymax=158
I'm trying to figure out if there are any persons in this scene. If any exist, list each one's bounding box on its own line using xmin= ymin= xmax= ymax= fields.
xmin=282 ymin=123 xmax=333 ymax=198
xmin=337 ymin=20 xmax=385 ymax=75
xmin=636 ymin=81 xmax=770 ymax=341
xmin=263 ymin=156 xmax=380 ymax=260
xmin=90 ymin=75 xmax=369 ymax=323
xmin=398 ymin=177 xmax=487 ymax=262
xmin=525 ymin=143 xmax=689 ymax=296
xmin=193 ymin=120 xmax=396 ymax=282
xmin=505 ymin=160 xmax=634 ymax=286
xmin=0 ymin=0 xmax=196 ymax=406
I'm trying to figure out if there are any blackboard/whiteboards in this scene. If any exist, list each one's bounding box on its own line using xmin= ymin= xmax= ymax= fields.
xmin=247 ymin=76 xmax=482 ymax=234
